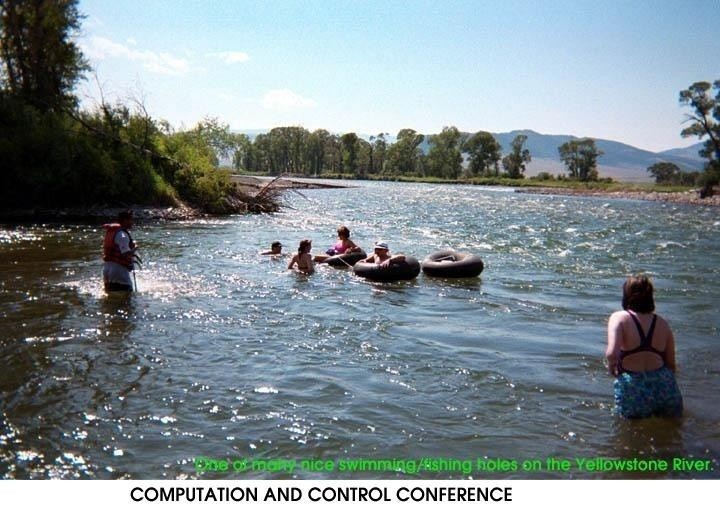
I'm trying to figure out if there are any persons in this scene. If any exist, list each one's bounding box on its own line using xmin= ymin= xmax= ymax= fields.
xmin=359 ymin=240 xmax=406 ymax=269
xmin=286 ymin=239 xmax=315 ymax=274
xmin=101 ymin=210 xmax=142 ymax=299
xmin=605 ymin=273 xmax=683 ymax=421
xmin=325 ymin=226 xmax=361 ymax=255
xmin=260 ymin=239 xmax=284 ymax=256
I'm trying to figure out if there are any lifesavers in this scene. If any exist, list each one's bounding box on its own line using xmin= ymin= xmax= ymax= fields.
xmin=321 ymin=249 xmax=367 ymax=266
xmin=353 ymin=255 xmax=421 ymax=282
xmin=420 ymin=249 xmax=484 ymax=278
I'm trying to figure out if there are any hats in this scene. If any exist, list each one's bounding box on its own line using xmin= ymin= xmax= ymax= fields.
xmin=375 ymin=242 xmax=388 ymax=250
